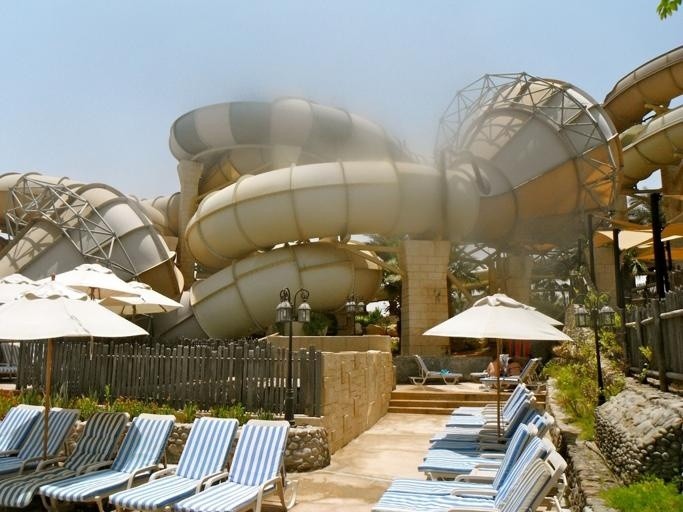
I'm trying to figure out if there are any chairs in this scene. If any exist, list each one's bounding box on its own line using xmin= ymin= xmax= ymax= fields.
xmin=0 ymin=401 xmax=298 ymax=512
xmin=369 ymin=382 xmax=568 ymax=512
xmin=406 ymin=354 xmax=543 ymax=392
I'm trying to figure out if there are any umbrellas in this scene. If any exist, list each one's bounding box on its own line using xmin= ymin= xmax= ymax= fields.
xmin=33 ymin=261 xmax=141 ymax=308
xmin=0 ymin=273 xmax=150 ymax=461
xmin=92 ymin=277 xmax=183 ymax=328
xmin=419 ymin=290 xmax=574 ymax=446
xmin=1 ymin=272 xmax=89 ymax=309
xmin=498 ymin=292 xmax=563 ymax=327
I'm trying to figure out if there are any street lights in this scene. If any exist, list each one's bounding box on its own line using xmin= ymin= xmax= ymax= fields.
xmin=573 ymin=294 xmax=616 ymax=404
xmin=274 ymin=285 xmax=312 ymax=428
xmin=344 ymin=295 xmax=366 ymax=334
